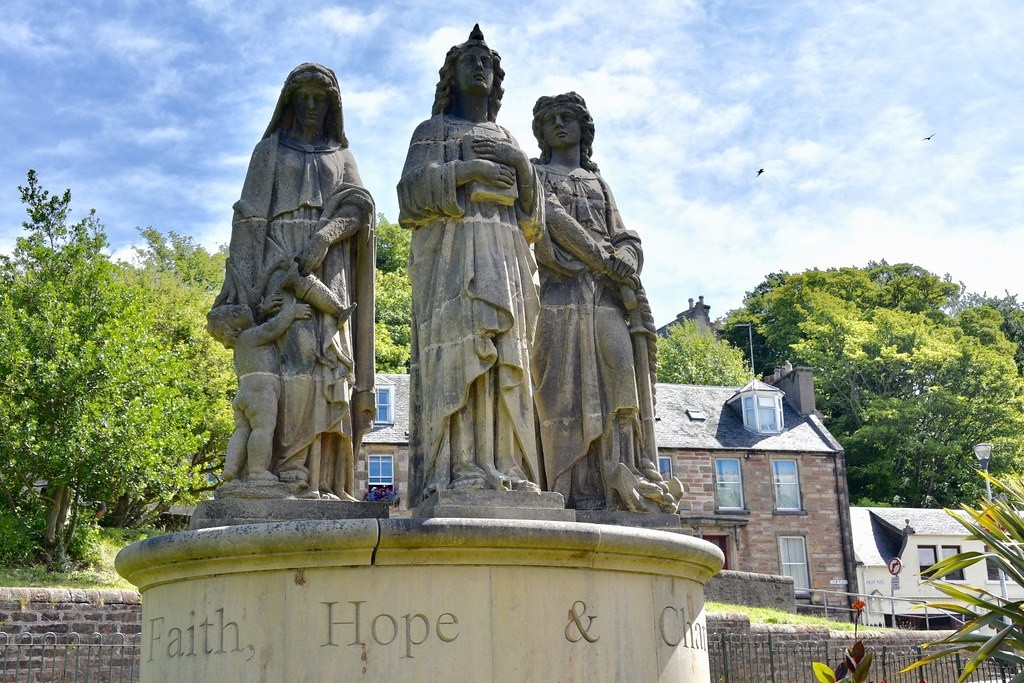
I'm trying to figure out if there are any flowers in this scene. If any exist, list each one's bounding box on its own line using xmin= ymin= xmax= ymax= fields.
xmin=363 ymin=486 xmax=401 ymax=507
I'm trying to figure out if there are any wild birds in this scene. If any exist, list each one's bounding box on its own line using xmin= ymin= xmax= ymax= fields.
xmin=756 ymin=168 xmax=764 ymax=177
xmin=922 ymin=134 xmax=936 ymax=141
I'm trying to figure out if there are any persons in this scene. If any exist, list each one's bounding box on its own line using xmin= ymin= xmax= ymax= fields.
xmin=396 ymin=22 xmax=545 ymax=494
xmin=525 ymin=92 xmax=678 ymax=517
xmin=205 ymin=62 xmax=376 ymax=503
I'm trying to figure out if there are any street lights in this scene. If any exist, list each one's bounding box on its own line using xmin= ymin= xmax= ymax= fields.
xmin=972 ymin=442 xmax=1015 ymax=640
